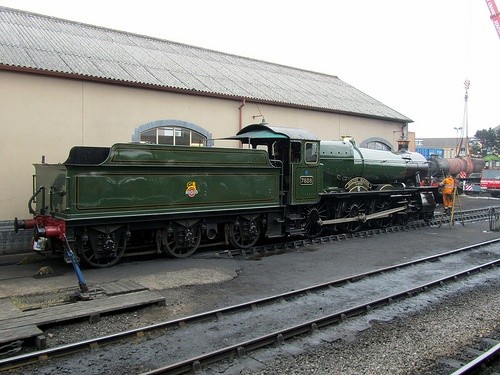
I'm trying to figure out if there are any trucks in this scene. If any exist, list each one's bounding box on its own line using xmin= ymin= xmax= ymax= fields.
xmin=480 ymin=166 xmax=500 ymax=197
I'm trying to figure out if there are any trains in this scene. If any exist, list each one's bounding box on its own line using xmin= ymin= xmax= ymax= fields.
xmin=14 ymin=125 xmax=439 ymax=268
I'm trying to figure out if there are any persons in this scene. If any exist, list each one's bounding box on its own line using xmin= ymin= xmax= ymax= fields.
xmin=439 ymin=173 xmax=455 ymax=213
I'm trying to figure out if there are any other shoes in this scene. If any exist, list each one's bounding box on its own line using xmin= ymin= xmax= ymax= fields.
xmin=444 ymin=206 xmax=451 ymax=213
